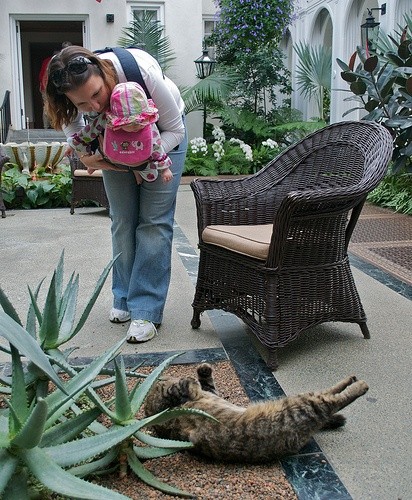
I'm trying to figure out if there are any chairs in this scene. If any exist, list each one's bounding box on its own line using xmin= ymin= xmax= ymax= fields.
xmin=190 ymin=120 xmax=393 ymax=372
xmin=67 ymin=153 xmax=110 ymax=216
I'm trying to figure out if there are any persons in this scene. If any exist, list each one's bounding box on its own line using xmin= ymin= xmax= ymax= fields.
xmin=38 ymin=42 xmax=78 ymax=130
xmin=64 ymin=81 xmax=175 ymax=184
xmin=42 ymin=46 xmax=188 ymax=344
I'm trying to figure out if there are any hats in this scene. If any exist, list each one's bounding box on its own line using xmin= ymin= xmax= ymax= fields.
xmin=106 ymin=82 xmax=159 ymax=130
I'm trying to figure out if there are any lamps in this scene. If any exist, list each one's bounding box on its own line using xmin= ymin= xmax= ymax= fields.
xmin=107 ymin=14 xmax=114 ymax=22
xmin=361 ymin=3 xmax=386 ymax=56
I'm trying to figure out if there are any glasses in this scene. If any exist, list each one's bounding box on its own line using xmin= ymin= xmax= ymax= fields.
xmin=50 ymin=56 xmax=95 ymax=88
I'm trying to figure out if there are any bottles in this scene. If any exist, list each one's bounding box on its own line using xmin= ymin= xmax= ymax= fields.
xmin=23 ymin=153 xmax=29 ymax=173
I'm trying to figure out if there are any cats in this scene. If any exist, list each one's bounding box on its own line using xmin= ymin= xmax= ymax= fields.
xmin=144 ymin=363 xmax=369 ymax=459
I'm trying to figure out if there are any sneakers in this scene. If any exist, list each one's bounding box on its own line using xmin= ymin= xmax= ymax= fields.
xmin=109 ymin=307 xmax=131 ymax=324
xmin=126 ymin=320 xmax=162 ymax=344
